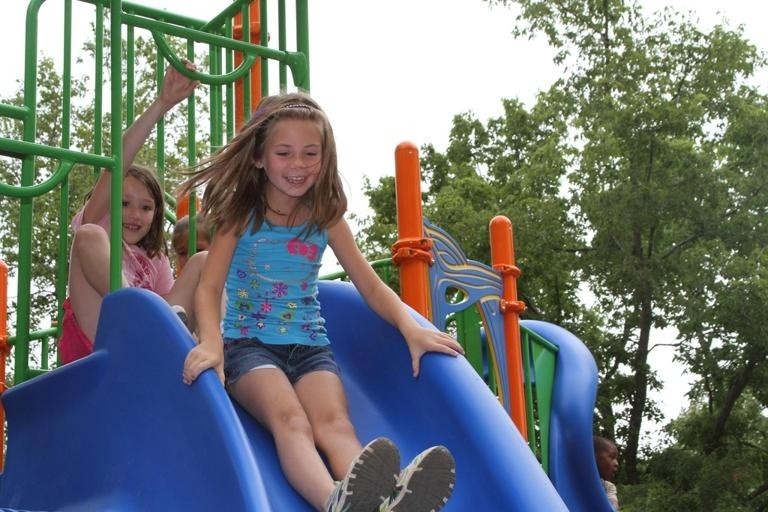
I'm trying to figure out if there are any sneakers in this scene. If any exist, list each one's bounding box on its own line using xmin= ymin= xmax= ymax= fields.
xmin=323 ymin=437 xmax=401 ymax=511
xmin=376 ymin=445 xmax=457 ymax=511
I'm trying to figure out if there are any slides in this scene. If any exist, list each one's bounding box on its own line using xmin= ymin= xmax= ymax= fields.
xmin=0 ymin=280 xmax=573 ymax=512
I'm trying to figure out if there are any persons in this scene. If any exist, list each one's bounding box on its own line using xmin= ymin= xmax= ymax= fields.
xmin=595 ymin=435 xmax=619 ymax=511
xmin=181 ymin=91 xmax=465 ymax=512
xmin=173 ymin=208 xmax=227 ymax=339
xmin=60 ymin=58 xmax=222 ymax=365
